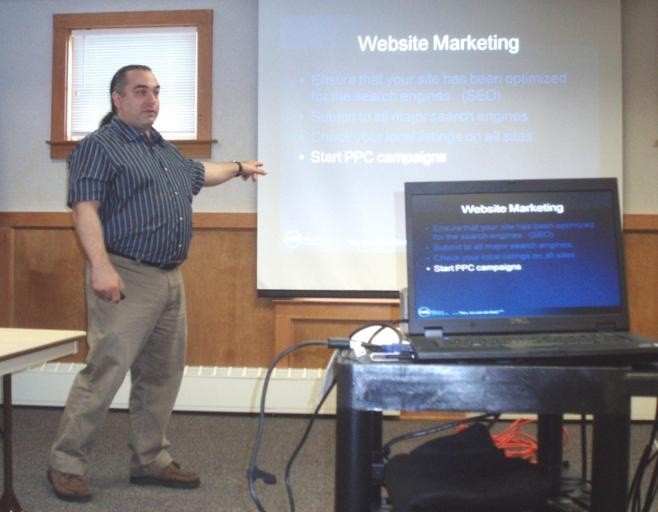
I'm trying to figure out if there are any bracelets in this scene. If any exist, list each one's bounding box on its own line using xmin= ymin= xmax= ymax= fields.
xmin=231 ymin=160 xmax=243 ymax=178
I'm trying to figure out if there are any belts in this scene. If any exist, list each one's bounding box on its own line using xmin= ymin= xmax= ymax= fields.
xmin=104 ymin=247 xmax=188 ymax=271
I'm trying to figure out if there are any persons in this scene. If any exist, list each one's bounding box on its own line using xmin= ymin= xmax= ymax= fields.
xmin=44 ymin=64 xmax=271 ymax=503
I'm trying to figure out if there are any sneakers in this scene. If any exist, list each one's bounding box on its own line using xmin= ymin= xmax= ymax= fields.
xmin=127 ymin=457 xmax=200 ymax=490
xmin=43 ymin=462 xmax=93 ymax=502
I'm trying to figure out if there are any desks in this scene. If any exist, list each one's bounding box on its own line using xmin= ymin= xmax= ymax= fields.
xmin=336 ymin=347 xmax=657 ymax=512
xmin=0 ymin=328 xmax=87 ymax=512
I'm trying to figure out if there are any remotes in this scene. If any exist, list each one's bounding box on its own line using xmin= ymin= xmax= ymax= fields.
xmin=119 ymin=291 xmax=126 ymax=301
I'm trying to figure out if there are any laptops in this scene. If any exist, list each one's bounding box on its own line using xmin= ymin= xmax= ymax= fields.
xmin=403 ymin=176 xmax=658 ymax=362
